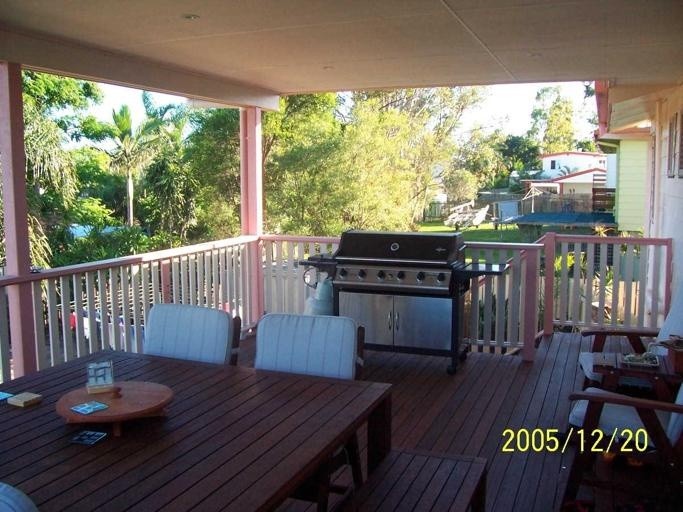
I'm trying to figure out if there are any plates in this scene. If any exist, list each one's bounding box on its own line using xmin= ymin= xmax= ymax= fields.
xmin=619 ymin=353 xmax=660 ymax=367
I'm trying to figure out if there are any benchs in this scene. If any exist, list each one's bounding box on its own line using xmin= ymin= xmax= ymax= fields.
xmin=337 ymin=447 xmax=488 ymax=510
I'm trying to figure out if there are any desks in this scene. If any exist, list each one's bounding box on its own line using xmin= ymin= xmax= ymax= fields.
xmin=0 ymin=348 xmax=393 ymax=510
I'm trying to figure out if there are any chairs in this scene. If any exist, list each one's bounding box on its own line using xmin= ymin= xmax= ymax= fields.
xmin=554 ymin=283 xmax=682 ymax=509
xmin=0 ymin=481 xmax=38 ymax=511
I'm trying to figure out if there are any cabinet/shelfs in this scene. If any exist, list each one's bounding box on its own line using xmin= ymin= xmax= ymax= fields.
xmin=297 ymin=227 xmax=509 ymax=377
